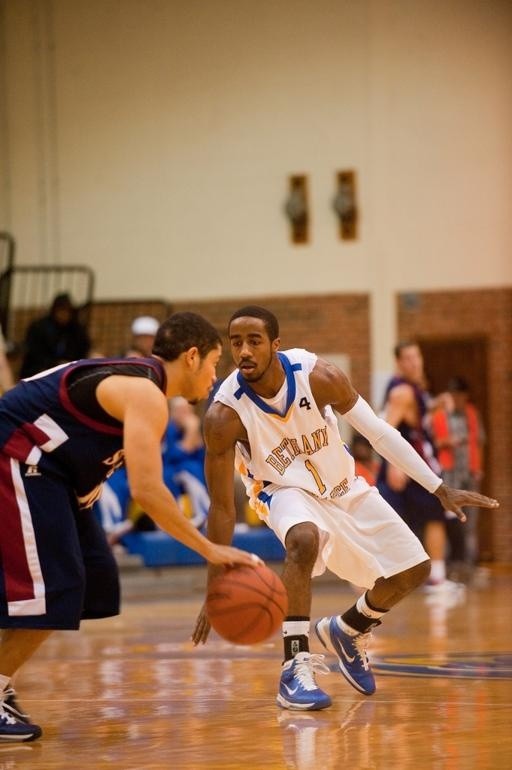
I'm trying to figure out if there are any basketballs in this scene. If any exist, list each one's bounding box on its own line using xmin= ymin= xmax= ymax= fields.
xmin=208 ymin=564 xmax=288 ymax=644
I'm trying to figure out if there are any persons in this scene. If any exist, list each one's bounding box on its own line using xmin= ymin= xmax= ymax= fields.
xmin=189 ymin=304 xmax=501 ymax=711
xmin=0 ymin=312 xmax=266 ymax=743
xmin=1 ymin=293 xmax=270 ymax=547
xmin=352 ymin=338 xmax=492 ymax=593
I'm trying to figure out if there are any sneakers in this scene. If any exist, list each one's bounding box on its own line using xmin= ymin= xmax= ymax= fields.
xmin=315 ymin=613 xmax=376 ymax=696
xmin=0 ymin=685 xmax=41 ymax=741
xmin=276 ymin=651 xmax=333 ymax=711
